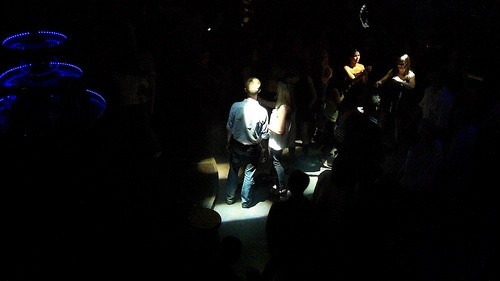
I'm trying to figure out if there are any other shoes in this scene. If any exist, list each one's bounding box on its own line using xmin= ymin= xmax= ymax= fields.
xmin=242 ymin=197 xmax=253 ymax=209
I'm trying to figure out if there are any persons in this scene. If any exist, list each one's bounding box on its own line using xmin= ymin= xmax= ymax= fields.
xmin=122 ymin=22 xmax=500 ymax=281
xmin=224 ymin=77 xmax=270 ymax=208
